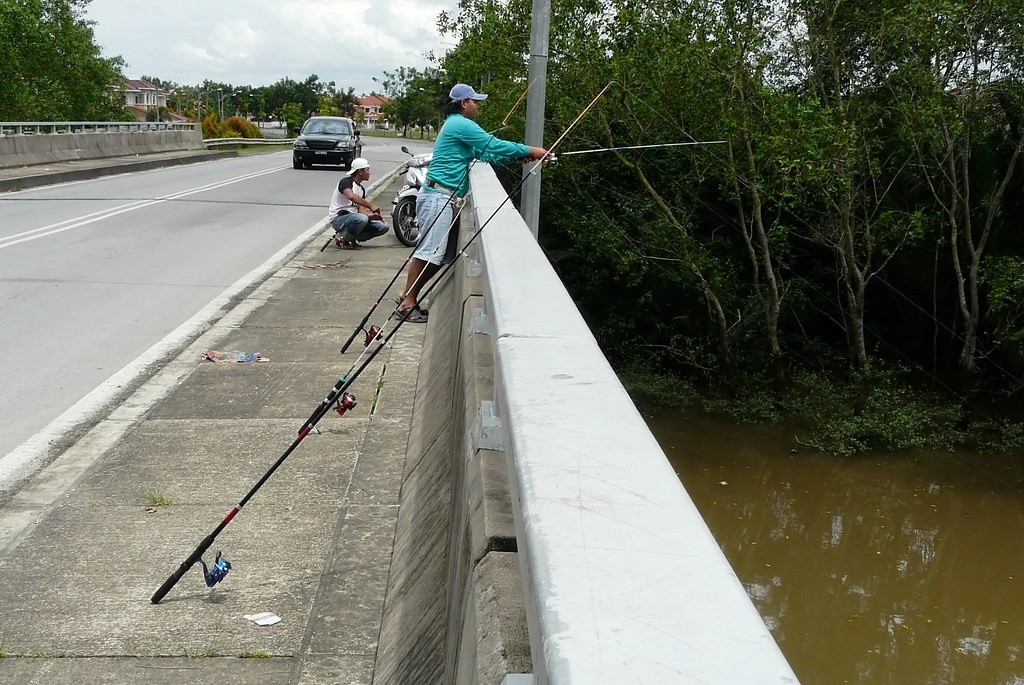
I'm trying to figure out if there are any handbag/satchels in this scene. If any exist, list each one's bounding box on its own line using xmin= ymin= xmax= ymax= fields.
xmin=428 ymin=181 xmax=462 ymax=216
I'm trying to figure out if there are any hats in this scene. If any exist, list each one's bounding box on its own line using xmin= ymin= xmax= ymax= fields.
xmin=449 ymin=84 xmax=489 ymax=101
xmin=346 ymin=157 xmax=369 ymax=176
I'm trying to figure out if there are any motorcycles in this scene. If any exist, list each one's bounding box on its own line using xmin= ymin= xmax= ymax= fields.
xmin=390 ymin=146 xmax=435 ymax=247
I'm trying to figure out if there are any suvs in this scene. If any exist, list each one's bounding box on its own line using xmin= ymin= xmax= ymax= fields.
xmin=292 ymin=116 xmax=362 ymax=171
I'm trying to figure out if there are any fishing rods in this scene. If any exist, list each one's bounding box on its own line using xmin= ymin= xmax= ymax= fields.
xmin=337 ymin=72 xmax=542 ymax=357
xmin=319 ymin=164 xmax=405 ymax=253
xmin=520 ymin=136 xmax=732 ymax=168
xmin=144 ymin=76 xmax=617 ymax=611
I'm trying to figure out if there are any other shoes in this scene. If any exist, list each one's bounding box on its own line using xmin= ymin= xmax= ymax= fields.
xmin=335 ymin=235 xmax=354 ymax=249
xmin=349 ymin=240 xmax=362 ymax=247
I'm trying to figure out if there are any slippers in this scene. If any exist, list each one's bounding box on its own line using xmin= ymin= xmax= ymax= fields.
xmin=396 ymin=308 xmax=428 ymax=323
xmin=394 ymin=297 xmax=428 ymax=316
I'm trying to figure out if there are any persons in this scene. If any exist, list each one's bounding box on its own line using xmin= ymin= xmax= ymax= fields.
xmin=329 ymin=158 xmax=389 ymax=250
xmin=394 ymin=83 xmax=553 ymax=325
